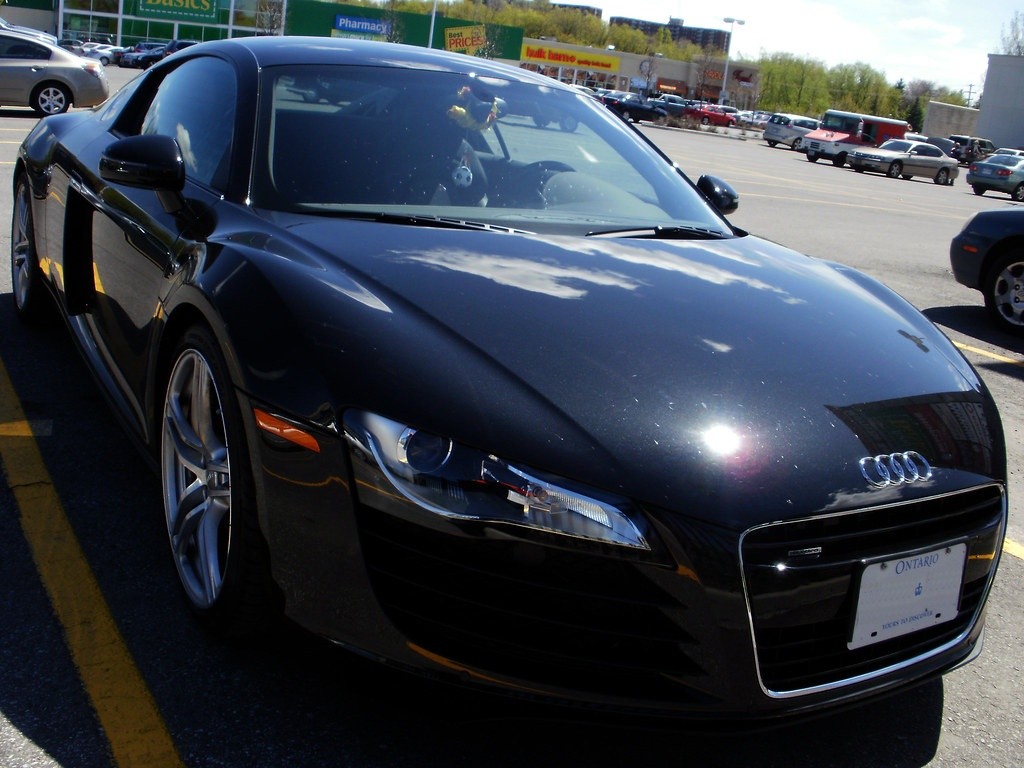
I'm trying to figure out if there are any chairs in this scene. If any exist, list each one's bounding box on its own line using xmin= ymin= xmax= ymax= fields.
xmin=363 ymin=89 xmax=488 ymax=207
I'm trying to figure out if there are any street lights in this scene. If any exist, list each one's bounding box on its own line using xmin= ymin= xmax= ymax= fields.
xmin=718 ymin=18 xmax=746 ymax=105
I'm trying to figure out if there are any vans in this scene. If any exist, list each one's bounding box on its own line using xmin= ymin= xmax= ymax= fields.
xmin=763 ymin=113 xmax=822 ymax=152
xmin=802 ymin=109 xmax=908 ymax=167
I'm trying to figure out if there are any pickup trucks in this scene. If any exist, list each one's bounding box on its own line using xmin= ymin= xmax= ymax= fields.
xmin=111 ymin=41 xmax=169 ymax=67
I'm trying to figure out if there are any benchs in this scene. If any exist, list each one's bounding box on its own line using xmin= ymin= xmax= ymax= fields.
xmin=274 ymin=109 xmax=384 ymax=205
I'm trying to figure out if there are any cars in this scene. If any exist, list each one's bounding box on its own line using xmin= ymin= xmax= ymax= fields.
xmin=0 ymin=17 xmax=57 ymax=46
xmin=647 ymin=93 xmax=689 ymax=118
xmin=570 ymin=84 xmax=667 ymax=126
xmin=684 ymin=103 xmax=736 ymax=127
xmin=716 ymin=105 xmax=741 ymax=124
xmin=846 ymin=138 xmax=959 ymax=185
xmin=949 ymin=206 xmax=1024 ymax=337
xmin=7 ymin=35 xmax=1009 ymax=728
xmin=736 ymin=108 xmax=773 ymax=130
xmin=121 ymin=47 xmax=164 ymax=69
xmin=966 ymin=147 xmax=1024 ymax=202
xmin=0 ymin=30 xmax=109 ymax=117
xmin=477 ymin=86 xmax=581 ymax=132
xmin=57 ymin=39 xmax=135 ymax=66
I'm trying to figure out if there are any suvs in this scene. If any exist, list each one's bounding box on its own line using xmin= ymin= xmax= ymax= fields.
xmin=135 ymin=39 xmax=199 ymax=70
xmin=949 ymin=133 xmax=996 ymax=166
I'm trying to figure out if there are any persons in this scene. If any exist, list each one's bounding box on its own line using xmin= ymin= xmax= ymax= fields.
xmin=966 ymin=141 xmax=984 ymax=166
xmin=951 ymin=143 xmax=962 ymax=160
